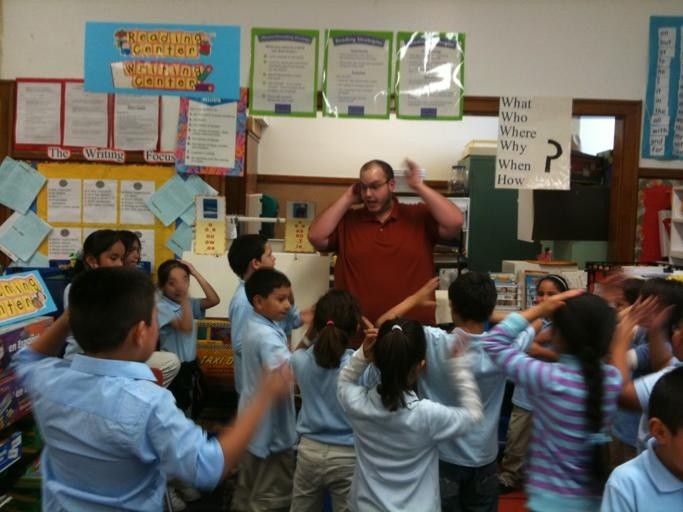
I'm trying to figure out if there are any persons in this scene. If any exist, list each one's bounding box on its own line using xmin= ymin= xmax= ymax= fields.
xmin=307 ymin=158 xmax=466 ymax=351
xmin=227 ymin=232 xmax=314 ymax=509
xmin=115 ymin=230 xmax=138 ymax=267
xmin=61 ymin=230 xmax=180 ymax=389
xmin=155 ymin=260 xmax=221 ymax=510
xmin=8 ymin=263 xmax=295 ymax=511
xmin=291 ymin=271 xmax=681 ymax=510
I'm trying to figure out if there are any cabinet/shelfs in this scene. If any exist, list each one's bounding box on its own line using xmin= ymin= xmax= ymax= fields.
xmin=0 ymin=316 xmax=54 ymax=510
xmin=665 ymin=182 xmax=683 ymax=264
